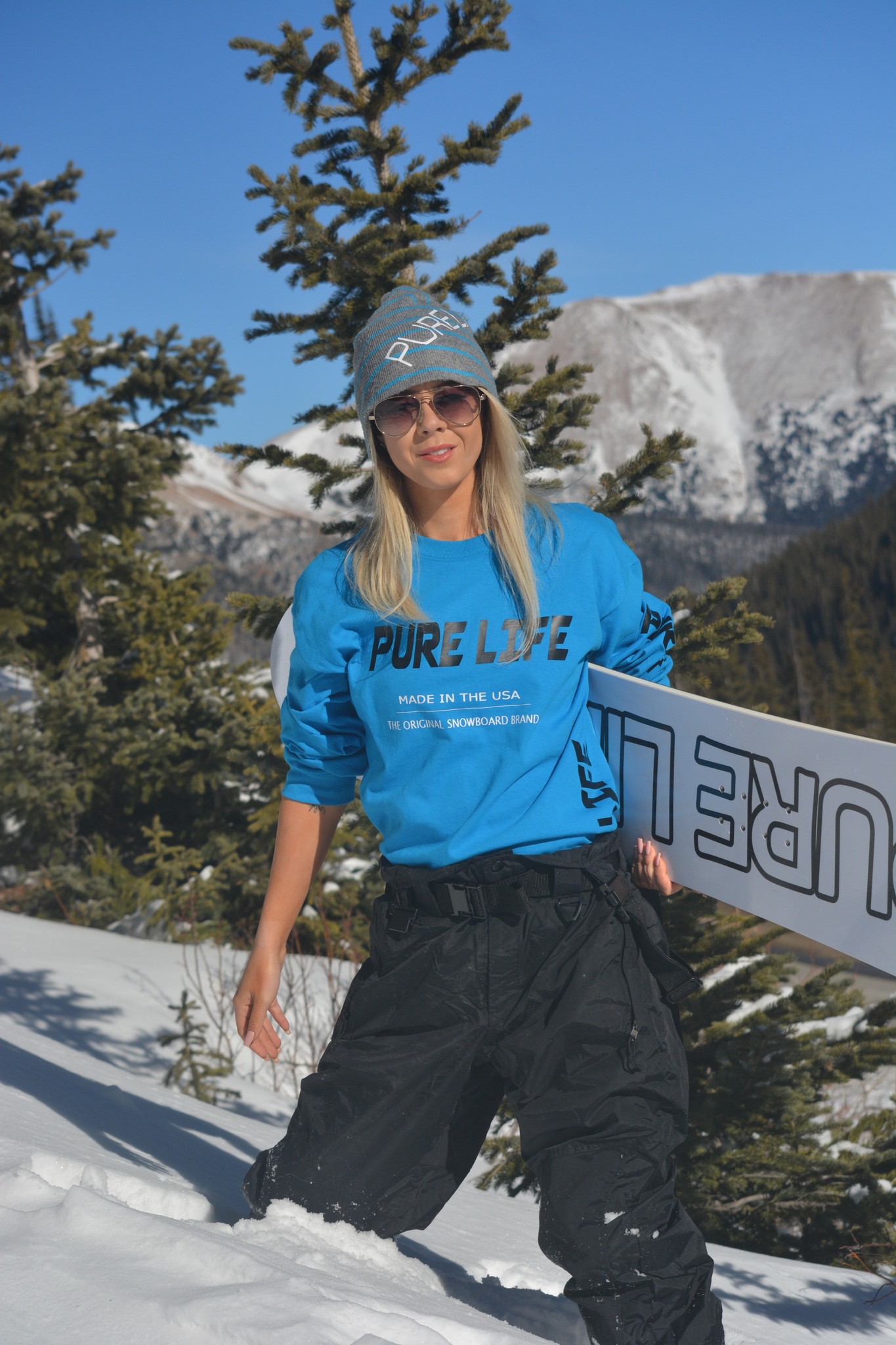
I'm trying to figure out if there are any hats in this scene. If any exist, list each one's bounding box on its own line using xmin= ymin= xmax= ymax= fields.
xmin=353 ymin=285 xmax=498 ymax=467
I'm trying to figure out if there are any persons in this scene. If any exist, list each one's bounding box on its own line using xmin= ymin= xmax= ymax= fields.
xmin=228 ymin=280 xmax=727 ymax=1345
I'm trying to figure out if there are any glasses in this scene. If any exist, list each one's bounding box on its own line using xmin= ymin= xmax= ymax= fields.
xmin=367 ymin=384 xmax=486 ymax=438
